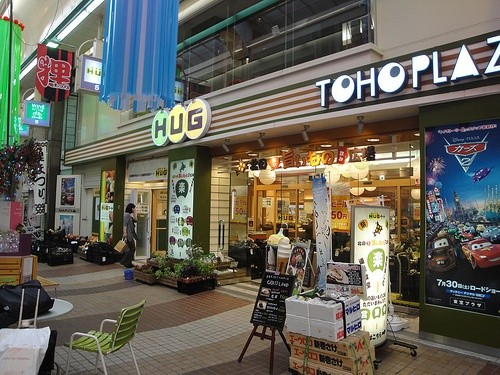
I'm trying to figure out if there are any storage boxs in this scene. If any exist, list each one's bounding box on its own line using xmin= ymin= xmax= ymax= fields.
xmin=289 ymin=356 xmax=373 ymax=375
xmin=287 ymin=330 xmax=370 ymax=358
xmin=0 ymin=326 xmax=51 ymax=375
xmin=284 ymin=289 xmax=362 ymax=342
xmin=291 ymin=345 xmax=375 ymax=373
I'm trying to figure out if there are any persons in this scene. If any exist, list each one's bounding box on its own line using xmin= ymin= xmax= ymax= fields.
xmin=121 ymin=203 xmax=138 ymax=268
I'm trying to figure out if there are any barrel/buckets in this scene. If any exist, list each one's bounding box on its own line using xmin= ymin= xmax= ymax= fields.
xmin=124 ymin=270 xmax=133 ymax=280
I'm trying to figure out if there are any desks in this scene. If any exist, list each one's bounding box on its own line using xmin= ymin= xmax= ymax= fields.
xmin=37 ymin=330 xmax=58 ymax=375
xmin=18 ymin=297 xmax=74 ymax=375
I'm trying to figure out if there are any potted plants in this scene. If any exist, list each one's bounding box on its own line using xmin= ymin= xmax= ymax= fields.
xmin=64 ymin=233 xmax=114 ymax=267
xmin=133 ymin=263 xmax=157 ymax=285
xmin=145 ymin=251 xmax=165 ymax=269
xmin=154 ymin=268 xmax=182 ymax=289
xmin=165 ymin=250 xmax=216 ymax=295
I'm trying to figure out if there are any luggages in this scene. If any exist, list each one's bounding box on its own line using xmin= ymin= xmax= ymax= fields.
xmin=16 ymin=285 xmax=57 ymax=375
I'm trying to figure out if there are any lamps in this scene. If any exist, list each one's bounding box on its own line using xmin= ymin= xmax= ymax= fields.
xmin=356 ymin=115 xmax=365 ymax=134
xmin=301 ymin=125 xmax=311 ymax=141
xmin=256 ymin=132 xmax=266 ymax=147
xmin=222 ymin=139 xmax=230 ymax=152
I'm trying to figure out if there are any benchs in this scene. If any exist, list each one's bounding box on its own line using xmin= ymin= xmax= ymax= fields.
xmin=36 ymin=275 xmax=60 ymax=299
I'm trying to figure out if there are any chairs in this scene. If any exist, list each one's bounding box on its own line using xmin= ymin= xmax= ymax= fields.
xmin=63 ymin=300 xmax=146 ymax=375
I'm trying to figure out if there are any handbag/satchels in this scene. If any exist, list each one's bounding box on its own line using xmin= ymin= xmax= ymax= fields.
xmin=0 ymin=280 xmax=55 ymax=328
xmin=114 ymin=237 xmax=127 ymax=253
